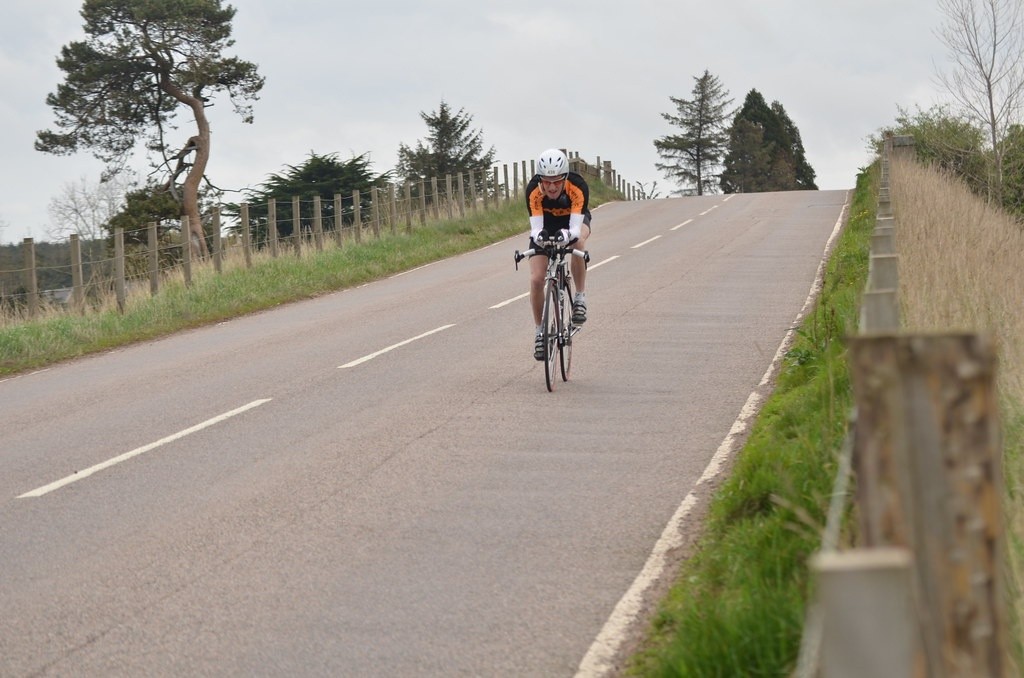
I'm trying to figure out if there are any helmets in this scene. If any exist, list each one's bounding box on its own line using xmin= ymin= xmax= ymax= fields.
xmin=536 ymin=149 xmax=570 ymax=180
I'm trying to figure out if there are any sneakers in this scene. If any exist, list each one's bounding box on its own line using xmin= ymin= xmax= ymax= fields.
xmin=534 ymin=332 xmax=546 ymax=360
xmin=572 ymin=301 xmax=586 ymax=323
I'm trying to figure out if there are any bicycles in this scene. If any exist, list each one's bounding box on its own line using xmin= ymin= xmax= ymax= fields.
xmin=515 ymin=234 xmax=590 ymax=392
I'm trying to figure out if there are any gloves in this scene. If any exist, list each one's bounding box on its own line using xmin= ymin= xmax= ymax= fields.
xmin=533 ymin=229 xmax=549 ymax=249
xmin=553 ymin=229 xmax=571 ymax=249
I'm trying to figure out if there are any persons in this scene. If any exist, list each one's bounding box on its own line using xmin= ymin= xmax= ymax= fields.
xmin=525 ymin=149 xmax=592 ymax=361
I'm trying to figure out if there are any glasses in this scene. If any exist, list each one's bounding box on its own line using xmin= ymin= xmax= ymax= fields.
xmin=540 ymin=177 xmax=565 ymax=186
